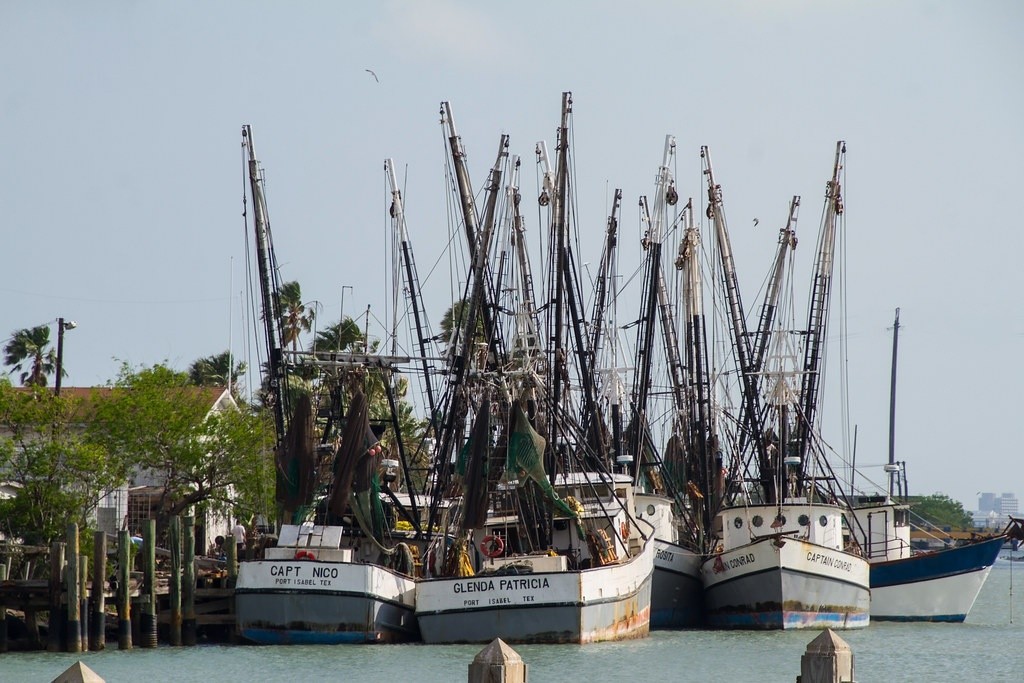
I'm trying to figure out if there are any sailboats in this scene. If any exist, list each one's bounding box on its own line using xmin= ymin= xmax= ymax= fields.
xmin=234 ymin=90 xmax=1024 ymax=647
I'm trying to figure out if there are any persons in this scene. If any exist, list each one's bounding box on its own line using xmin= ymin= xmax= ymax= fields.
xmin=230 ymin=519 xmax=246 ymax=550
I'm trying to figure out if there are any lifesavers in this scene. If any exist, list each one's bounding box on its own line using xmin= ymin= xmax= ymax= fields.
xmin=481 ymin=534 xmax=503 ymax=557
xmin=621 ymin=521 xmax=629 ymax=539
xmin=295 ymin=550 xmax=317 ymax=561
